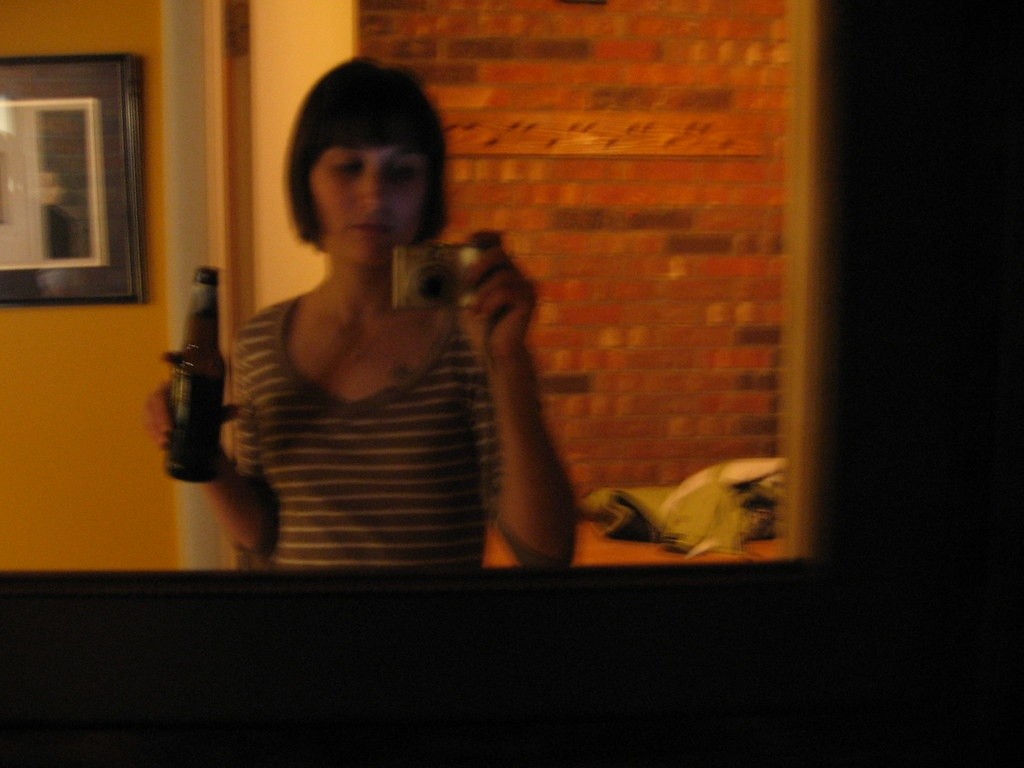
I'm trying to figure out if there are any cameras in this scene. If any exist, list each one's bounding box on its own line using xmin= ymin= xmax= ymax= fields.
xmin=391 ymin=244 xmax=489 ymax=309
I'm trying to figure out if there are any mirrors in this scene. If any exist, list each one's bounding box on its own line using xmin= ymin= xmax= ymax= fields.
xmin=0 ymin=1 xmax=828 ymax=592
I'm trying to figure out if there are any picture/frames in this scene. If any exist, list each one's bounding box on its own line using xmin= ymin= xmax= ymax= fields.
xmin=0 ymin=54 xmax=149 ymax=306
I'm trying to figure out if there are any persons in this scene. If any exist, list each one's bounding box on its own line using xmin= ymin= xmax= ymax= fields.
xmin=142 ymin=61 xmax=577 ymax=570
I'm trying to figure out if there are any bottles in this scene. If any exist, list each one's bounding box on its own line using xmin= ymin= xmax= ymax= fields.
xmin=163 ymin=266 xmax=228 ymax=479
